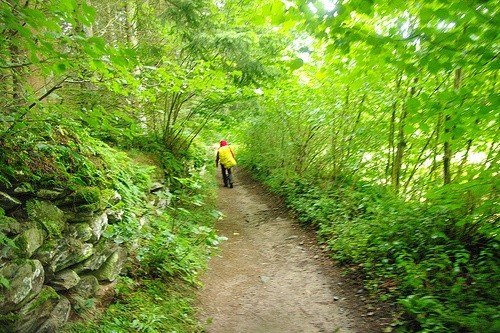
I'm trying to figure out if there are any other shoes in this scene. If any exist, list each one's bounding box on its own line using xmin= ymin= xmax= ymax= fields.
xmin=223 ymin=182 xmax=233 ymax=188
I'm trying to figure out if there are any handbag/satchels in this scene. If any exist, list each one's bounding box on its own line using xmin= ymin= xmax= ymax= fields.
xmin=219 ymin=145 xmax=237 ymax=169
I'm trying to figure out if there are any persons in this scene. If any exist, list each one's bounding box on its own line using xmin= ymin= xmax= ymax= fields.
xmin=216 ymin=140 xmax=235 ymax=189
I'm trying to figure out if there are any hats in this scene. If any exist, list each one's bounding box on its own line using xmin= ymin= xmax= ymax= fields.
xmin=220 ymin=141 xmax=227 ymax=146
